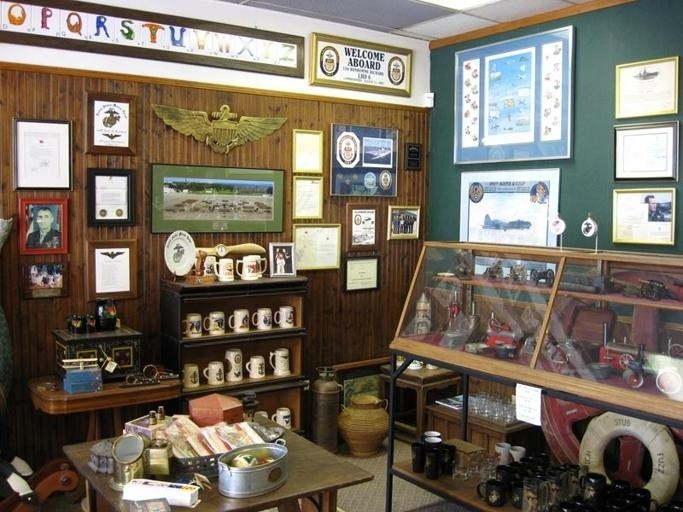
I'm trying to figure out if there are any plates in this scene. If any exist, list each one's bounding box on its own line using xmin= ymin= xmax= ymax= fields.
xmin=406 ymin=365 xmax=423 ymax=370
xmin=164 ymin=230 xmax=196 ymax=277
xmin=426 ymin=364 xmax=438 ymax=370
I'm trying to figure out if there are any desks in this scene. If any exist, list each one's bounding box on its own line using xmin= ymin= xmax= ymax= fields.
xmin=23 ymin=365 xmax=183 ymax=442
xmin=63 ymin=412 xmax=374 ymax=512
xmin=380 ymin=363 xmax=463 ymax=445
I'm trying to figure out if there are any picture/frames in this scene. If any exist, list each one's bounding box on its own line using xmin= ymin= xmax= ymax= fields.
xmin=459 ymin=167 xmax=560 ymax=283
xmin=13 ymin=90 xmax=138 ymax=302
xmin=292 ymin=122 xmax=422 ymax=294
xmin=454 ymin=25 xmax=575 ymax=166
xmin=269 ymin=242 xmax=297 ymax=277
xmin=309 ymin=31 xmax=413 ymax=97
xmin=613 ymin=121 xmax=680 ymax=185
xmin=0 ymin=0 xmax=305 ymax=77
xmin=613 ymin=57 xmax=679 ymax=119
xmin=612 ymin=187 xmax=676 ymax=245
xmin=149 ymin=161 xmax=286 ymax=234
xmin=332 ymin=357 xmax=406 ymax=444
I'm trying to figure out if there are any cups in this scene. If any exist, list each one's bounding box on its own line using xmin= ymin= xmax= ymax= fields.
xmin=194 ymin=254 xmax=268 ymax=282
xmin=181 ymin=304 xmax=296 ymax=338
xmin=271 ymin=407 xmax=292 ymax=431
xmin=396 ymin=355 xmax=407 ymax=366
xmin=254 ymin=410 xmax=269 ymax=420
xmin=410 ymin=359 xmax=424 ymax=366
xmin=63 ymin=312 xmax=97 ymax=335
xmin=180 ymin=346 xmax=292 ymax=390
xmin=468 ymin=391 xmax=520 ymax=425
xmin=405 ymin=425 xmax=681 ymax=511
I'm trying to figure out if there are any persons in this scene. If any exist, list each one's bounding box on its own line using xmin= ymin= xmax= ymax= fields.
xmin=282 ymin=248 xmax=291 ymax=273
xmin=273 ymin=247 xmax=280 ymax=271
xmin=25 ymin=205 xmax=62 ymax=248
xmin=391 ymin=211 xmax=416 ymax=236
xmin=643 ymin=194 xmax=671 ymax=222
xmin=275 ymin=253 xmax=286 ymax=272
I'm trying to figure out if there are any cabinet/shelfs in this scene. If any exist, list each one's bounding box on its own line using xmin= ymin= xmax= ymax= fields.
xmin=159 ymin=276 xmax=308 ymax=435
xmin=385 ymin=241 xmax=683 ymax=512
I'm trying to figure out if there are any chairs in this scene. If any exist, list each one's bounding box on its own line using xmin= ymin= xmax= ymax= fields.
xmin=0 ymin=303 xmax=80 ymax=512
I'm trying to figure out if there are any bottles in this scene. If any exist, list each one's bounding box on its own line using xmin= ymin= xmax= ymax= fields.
xmin=143 ymin=439 xmax=175 ymax=482
xmin=156 ymin=406 xmax=166 ymax=421
xmin=149 ymin=410 xmax=157 ymax=424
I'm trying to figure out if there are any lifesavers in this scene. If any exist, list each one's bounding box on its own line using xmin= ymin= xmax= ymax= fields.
xmin=579 ymin=410 xmax=680 ymax=511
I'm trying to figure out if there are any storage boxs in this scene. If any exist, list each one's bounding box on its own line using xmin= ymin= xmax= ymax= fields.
xmin=423 ymin=397 xmax=545 ymax=457
xmin=52 ymin=324 xmax=144 ymax=381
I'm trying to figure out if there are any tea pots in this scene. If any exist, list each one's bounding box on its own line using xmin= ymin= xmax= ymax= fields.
xmin=86 ymin=298 xmax=118 ymax=332
